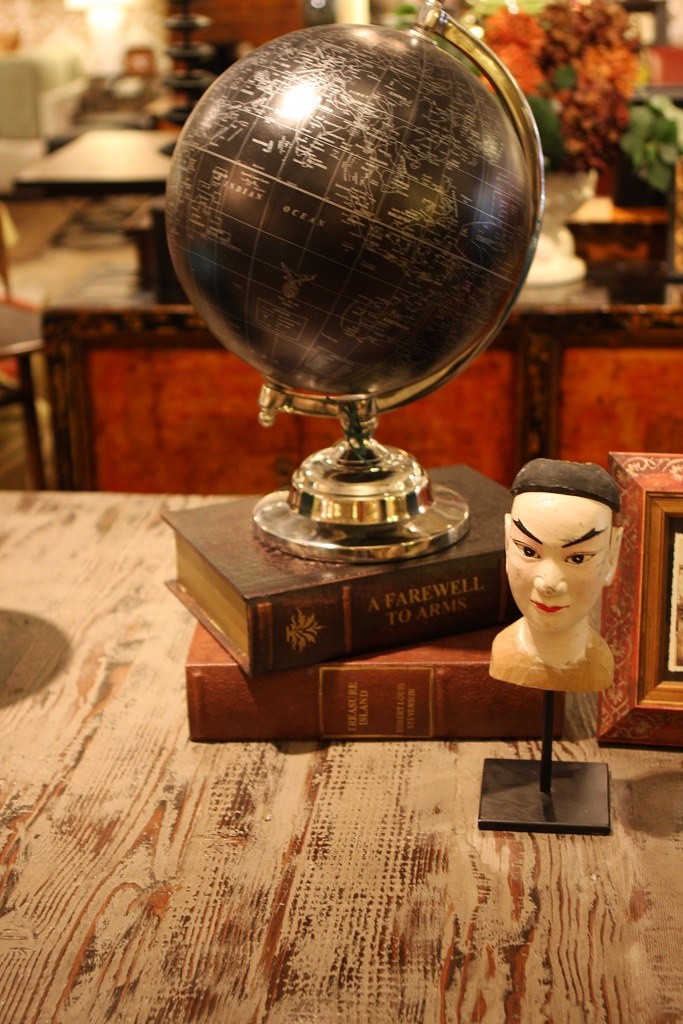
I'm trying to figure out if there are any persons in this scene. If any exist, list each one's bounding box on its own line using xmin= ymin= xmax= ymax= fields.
xmin=488 ymin=458 xmax=624 ymax=692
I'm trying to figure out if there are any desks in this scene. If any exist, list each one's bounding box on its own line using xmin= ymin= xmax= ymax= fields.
xmin=0 ymin=491 xmax=683 ymax=1024
xmin=0 ymin=127 xmax=175 ymax=198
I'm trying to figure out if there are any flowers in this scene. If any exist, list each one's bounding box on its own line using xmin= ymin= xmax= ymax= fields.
xmin=538 ymin=0 xmax=683 ymax=193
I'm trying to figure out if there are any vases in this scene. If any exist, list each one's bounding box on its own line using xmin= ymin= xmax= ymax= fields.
xmin=563 ymin=159 xmax=671 ymax=283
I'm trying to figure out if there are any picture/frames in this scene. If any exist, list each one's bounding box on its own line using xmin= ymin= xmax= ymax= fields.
xmin=594 ymin=452 xmax=683 ymax=748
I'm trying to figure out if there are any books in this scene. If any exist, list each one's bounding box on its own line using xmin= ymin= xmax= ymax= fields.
xmin=158 ymin=462 xmax=512 ymax=675
xmin=185 ymin=624 xmax=566 ymax=740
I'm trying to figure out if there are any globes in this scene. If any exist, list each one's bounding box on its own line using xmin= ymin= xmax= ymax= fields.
xmin=160 ymin=1 xmax=549 ymax=566
